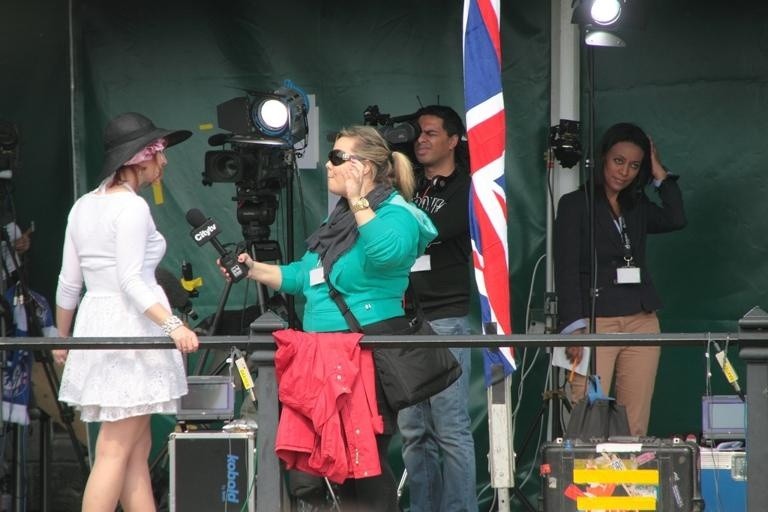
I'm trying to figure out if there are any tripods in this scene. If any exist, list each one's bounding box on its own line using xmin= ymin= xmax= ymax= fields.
xmin=0 ymin=228 xmax=52 ymax=512
xmin=492 ymin=156 xmax=571 ymax=512
xmin=196 ymin=239 xmax=303 ymax=375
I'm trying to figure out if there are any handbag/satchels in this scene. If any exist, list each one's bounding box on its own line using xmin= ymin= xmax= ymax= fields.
xmin=357 ymin=311 xmax=462 ymax=410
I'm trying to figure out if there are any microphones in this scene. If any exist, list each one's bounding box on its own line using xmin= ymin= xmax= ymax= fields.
xmin=185 ymin=208 xmax=230 ymax=258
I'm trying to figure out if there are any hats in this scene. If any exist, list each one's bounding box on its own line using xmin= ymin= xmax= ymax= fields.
xmin=96 ymin=111 xmax=194 ymax=173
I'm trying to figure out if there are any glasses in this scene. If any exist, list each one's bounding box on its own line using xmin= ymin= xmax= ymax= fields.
xmin=327 ymin=149 xmax=366 ymax=167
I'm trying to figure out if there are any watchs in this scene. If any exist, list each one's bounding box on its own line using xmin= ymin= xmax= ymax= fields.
xmin=351 ymin=198 xmax=370 ymax=214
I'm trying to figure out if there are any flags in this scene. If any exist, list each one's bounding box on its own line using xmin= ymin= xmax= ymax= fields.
xmin=461 ymin=0 xmax=519 ymax=388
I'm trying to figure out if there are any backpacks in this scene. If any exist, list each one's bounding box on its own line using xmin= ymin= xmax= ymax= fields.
xmin=568 ymin=378 xmax=631 ymax=444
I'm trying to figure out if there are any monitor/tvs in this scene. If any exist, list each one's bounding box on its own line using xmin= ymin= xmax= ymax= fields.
xmin=176 ymin=375 xmax=235 ymax=421
xmin=702 ymin=395 xmax=747 ymax=440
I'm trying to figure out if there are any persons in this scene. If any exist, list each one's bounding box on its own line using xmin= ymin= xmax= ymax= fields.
xmin=216 ymin=123 xmax=438 ymax=512
xmin=553 ymin=121 xmax=689 ymax=437
xmin=397 ymin=104 xmax=479 ymax=512
xmin=50 ymin=112 xmax=199 ymax=512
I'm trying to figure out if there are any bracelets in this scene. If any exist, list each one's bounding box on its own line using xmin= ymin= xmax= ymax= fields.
xmin=160 ymin=315 xmax=184 ymax=335
